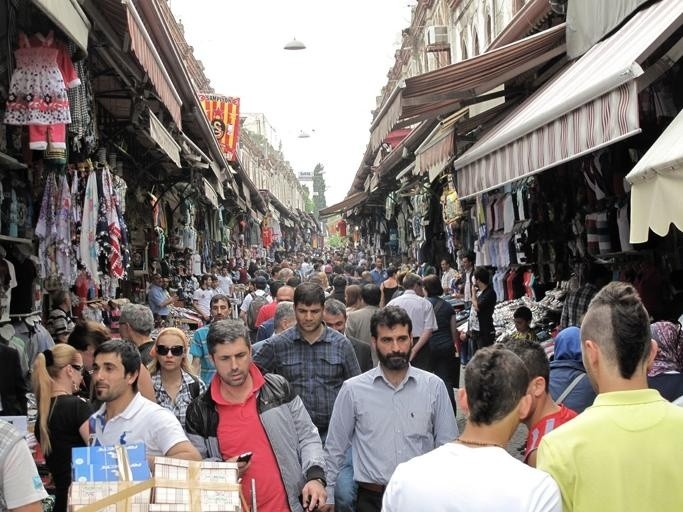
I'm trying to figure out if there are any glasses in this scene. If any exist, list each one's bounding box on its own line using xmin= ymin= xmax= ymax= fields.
xmin=71 ymin=364 xmax=85 ymax=377
xmin=156 ymin=344 xmax=184 ymax=357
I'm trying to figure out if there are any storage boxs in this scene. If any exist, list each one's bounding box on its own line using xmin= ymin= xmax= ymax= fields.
xmin=66 ymin=480 xmax=151 ymax=512
xmin=66 ymin=441 xmax=149 ymax=483
xmin=149 ymin=456 xmax=240 ymax=506
xmin=146 ymin=499 xmax=237 ymax=511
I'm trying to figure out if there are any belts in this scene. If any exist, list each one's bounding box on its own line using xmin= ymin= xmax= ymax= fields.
xmin=356 ymin=480 xmax=386 ymax=495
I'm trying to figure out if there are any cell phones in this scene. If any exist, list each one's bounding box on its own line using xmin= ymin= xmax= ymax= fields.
xmin=238 ymin=450 xmax=254 ymax=463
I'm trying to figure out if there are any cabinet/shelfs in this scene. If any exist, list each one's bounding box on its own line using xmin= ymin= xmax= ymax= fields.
xmin=0 ymin=149 xmax=36 ymax=245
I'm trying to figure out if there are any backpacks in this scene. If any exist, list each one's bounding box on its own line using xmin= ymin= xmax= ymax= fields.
xmin=247 ymin=292 xmax=270 ymax=330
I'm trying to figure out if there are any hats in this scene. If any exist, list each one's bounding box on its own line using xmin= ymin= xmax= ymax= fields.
xmin=251 ymin=276 xmax=266 ymax=285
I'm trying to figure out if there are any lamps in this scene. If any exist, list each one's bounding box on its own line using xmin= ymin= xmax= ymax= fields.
xmin=283 ymin=35 xmax=306 ymax=51
xmin=296 ymin=130 xmax=310 ymax=139
